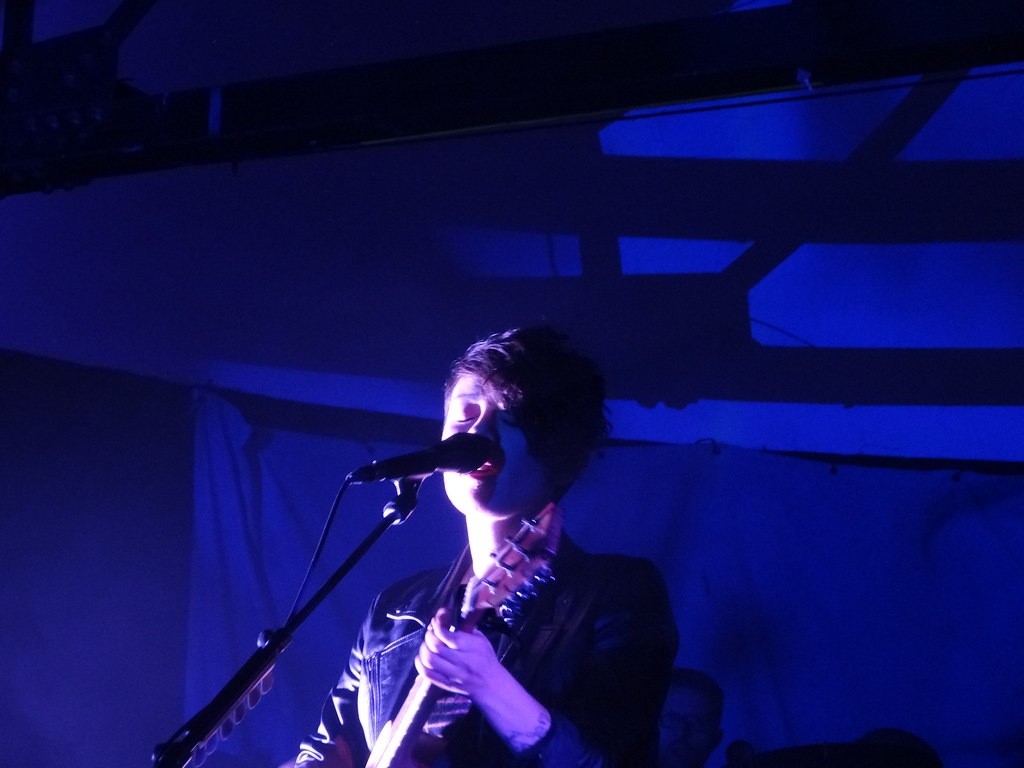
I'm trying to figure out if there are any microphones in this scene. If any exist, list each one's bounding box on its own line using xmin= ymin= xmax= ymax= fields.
xmin=345 ymin=432 xmax=493 ymax=484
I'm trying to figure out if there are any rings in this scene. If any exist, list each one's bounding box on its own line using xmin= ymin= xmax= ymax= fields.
xmin=447 ymin=677 xmax=464 ymax=688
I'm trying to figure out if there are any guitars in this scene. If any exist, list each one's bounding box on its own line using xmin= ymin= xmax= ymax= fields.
xmin=364 ymin=502 xmax=564 ymax=768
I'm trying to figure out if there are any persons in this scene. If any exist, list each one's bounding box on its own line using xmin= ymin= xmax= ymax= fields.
xmin=656 ymin=669 xmax=726 ymax=768
xmin=294 ymin=326 xmax=680 ymax=768
